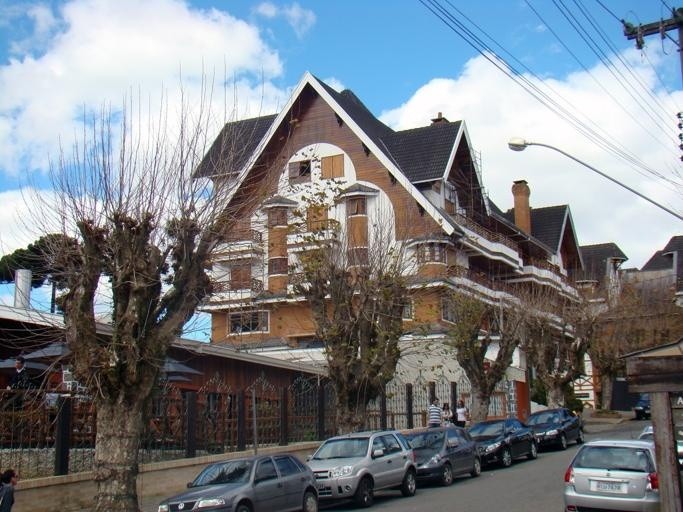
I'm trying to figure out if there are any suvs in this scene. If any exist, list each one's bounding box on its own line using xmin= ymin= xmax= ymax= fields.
xmin=305 ymin=428 xmax=417 ymax=511
xmin=562 ymin=440 xmax=660 ymax=512
xmin=635 ymin=423 xmax=682 ymax=472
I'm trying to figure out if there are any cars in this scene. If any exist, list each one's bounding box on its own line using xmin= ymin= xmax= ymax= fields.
xmin=522 ymin=407 xmax=584 ymax=449
xmin=402 ymin=428 xmax=481 ymax=487
xmin=156 ymin=452 xmax=319 ymax=512
xmin=634 ymin=394 xmax=650 ymax=420
xmin=467 ymin=417 xmax=536 ymax=469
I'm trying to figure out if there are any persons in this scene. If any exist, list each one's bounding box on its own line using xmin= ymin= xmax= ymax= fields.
xmin=427 ymin=395 xmax=469 ymax=428
xmin=0 ymin=468 xmax=17 ymax=512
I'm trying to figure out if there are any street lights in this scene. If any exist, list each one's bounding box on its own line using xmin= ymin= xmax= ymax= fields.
xmin=502 ymin=135 xmax=683 ymax=221
